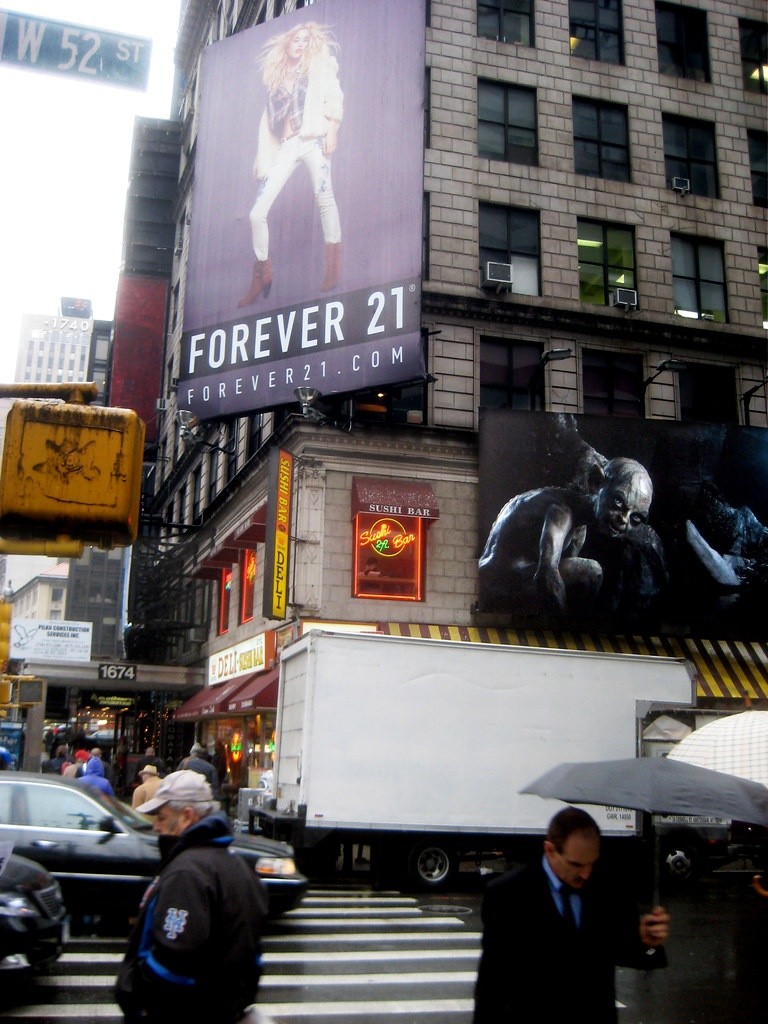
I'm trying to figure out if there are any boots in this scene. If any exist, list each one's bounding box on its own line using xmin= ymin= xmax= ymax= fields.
xmin=322 ymin=241 xmax=343 ymax=291
xmin=237 ymin=259 xmax=272 ymax=308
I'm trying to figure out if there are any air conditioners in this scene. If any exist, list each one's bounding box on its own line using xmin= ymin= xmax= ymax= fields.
xmin=613 ymin=288 xmax=637 ymax=308
xmin=174 ymin=241 xmax=183 ymax=256
xmin=156 ymin=398 xmax=169 ymax=410
xmin=671 ymin=177 xmax=690 ymax=192
xmin=482 ymin=261 xmax=514 ymax=289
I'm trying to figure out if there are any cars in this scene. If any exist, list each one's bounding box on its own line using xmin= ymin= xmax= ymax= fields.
xmin=1 ymin=770 xmax=309 ymax=920
xmin=86 ymin=730 xmax=130 ymax=747
xmin=0 ymin=844 xmax=70 ymax=989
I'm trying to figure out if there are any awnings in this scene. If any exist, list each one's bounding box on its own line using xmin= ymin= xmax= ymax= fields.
xmin=176 ymin=672 xmax=257 ymax=720
xmin=229 ymin=671 xmax=279 ymax=712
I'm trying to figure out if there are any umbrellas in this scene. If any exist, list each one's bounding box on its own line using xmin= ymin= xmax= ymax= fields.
xmin=554 ymin=755 xmax=768 ymax=939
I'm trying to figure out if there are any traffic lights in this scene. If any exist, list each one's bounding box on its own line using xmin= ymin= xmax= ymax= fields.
xmin=1 ymin=595 xmax=12 ymax=664
xmin=18 ymin=677 xmax=44 ymax=705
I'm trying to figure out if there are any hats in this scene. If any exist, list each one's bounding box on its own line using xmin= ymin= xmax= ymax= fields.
xmin=75 ymin=750 xmax=90 ymax=762
xmin=139 ymin=765 xmax=158 ymax=776
xmin=136 ymin=769 xmax=211 ymax=813
xmin=190 ymin=743 xmax=201 ymax=753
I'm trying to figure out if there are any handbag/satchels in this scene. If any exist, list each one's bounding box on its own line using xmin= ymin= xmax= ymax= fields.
xmin=43 ymin=738 xmax=47 ymax=743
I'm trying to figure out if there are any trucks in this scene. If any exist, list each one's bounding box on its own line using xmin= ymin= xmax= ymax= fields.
xmin=247 ymin=630 xmax=731 ymax=895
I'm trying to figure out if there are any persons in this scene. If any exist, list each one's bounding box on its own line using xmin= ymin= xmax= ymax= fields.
xmin=135 ymin=747 xmax=165 ymax=783
xmin=237 ymin=22 xmax=344 ymax=306
xmin=132 ymin=765 xmax=163 ymax=824
xmin=113 ymin=770 xmax=269 ymax=1024
xmin=479 ymin=458 xmax=654 ymax=615
xmin=43 ymin=726 xmax=73 ymax=759
xmin=176 ymin=741 xmax=226 ymax=801
xmin=473 ymin=808 xmax=671 ymax=1024
xmin=44 ymin=745 xmax=114 ymax=797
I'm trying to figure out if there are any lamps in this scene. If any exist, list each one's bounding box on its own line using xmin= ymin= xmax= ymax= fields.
xmin=175 ymin=410 xmax=238 ymax=455
xmin=293 ymin=387 xmax=353 ymax=433
xmin=644 ymin=358 xmax=686 ymax=389
xmin=540 ymin=348 xmax=573 ymax=368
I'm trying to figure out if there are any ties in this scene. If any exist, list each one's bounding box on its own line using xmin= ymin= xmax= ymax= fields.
xmin=558 ymin=885 xmax=576 ymax=930
